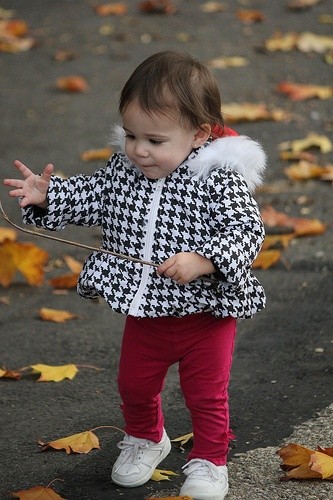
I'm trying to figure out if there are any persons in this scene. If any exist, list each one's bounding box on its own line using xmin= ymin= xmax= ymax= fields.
xmin=2 ymin=51 xmax=266 ymax=500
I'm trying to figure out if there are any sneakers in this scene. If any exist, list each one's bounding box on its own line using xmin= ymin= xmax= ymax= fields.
xmin=179 ymin=458 xmax=229 ymax=500
xmin=111 ymin=426 xmax=171 ymax=487
